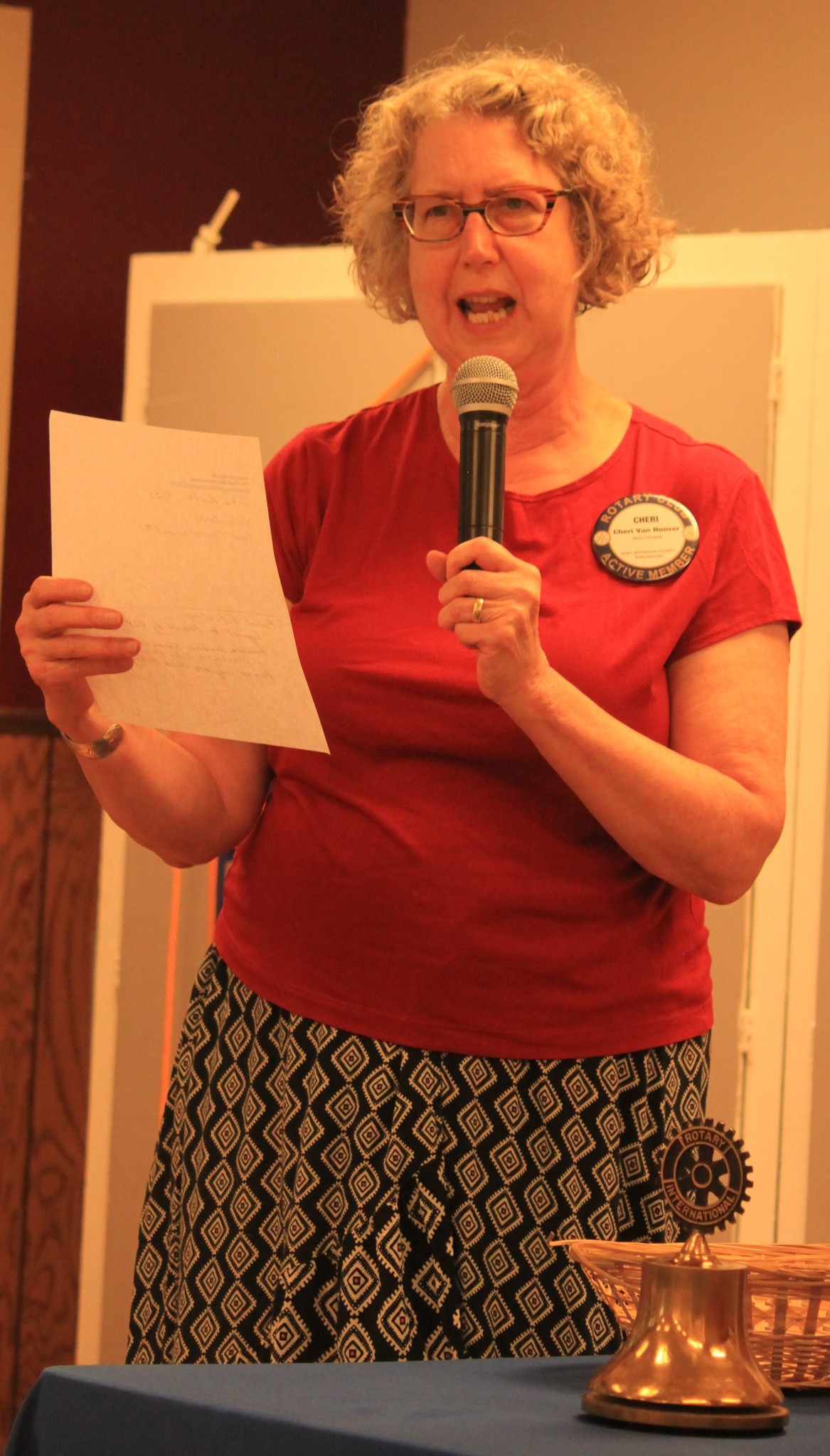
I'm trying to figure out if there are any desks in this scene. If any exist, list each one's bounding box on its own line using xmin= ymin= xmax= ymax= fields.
xmin=6 ymin=1355 xmax=830 ymax=1456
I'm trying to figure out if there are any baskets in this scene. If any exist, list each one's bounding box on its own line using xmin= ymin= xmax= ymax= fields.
xmin=548 ymin=1237 xmax=830 ymax=1393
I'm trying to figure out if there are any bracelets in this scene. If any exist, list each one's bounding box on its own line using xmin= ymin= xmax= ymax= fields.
xmin=60 ymin=723 xmax=124 ymax=760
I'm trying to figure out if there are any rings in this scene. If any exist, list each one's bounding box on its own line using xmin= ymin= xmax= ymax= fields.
xmin=472 ymin=598 xmax=484 ymax=623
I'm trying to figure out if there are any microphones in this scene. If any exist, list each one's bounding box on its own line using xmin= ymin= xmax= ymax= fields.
xmin=451 ymin=355 xmax=520 ymax=573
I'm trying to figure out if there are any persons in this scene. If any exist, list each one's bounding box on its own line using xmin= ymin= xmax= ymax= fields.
xmin=10 ymin=43 xmax=807 ymax=1366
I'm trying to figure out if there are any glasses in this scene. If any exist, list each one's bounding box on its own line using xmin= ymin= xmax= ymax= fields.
xmin=391 ymin=186 xmax=574 ymax=244
